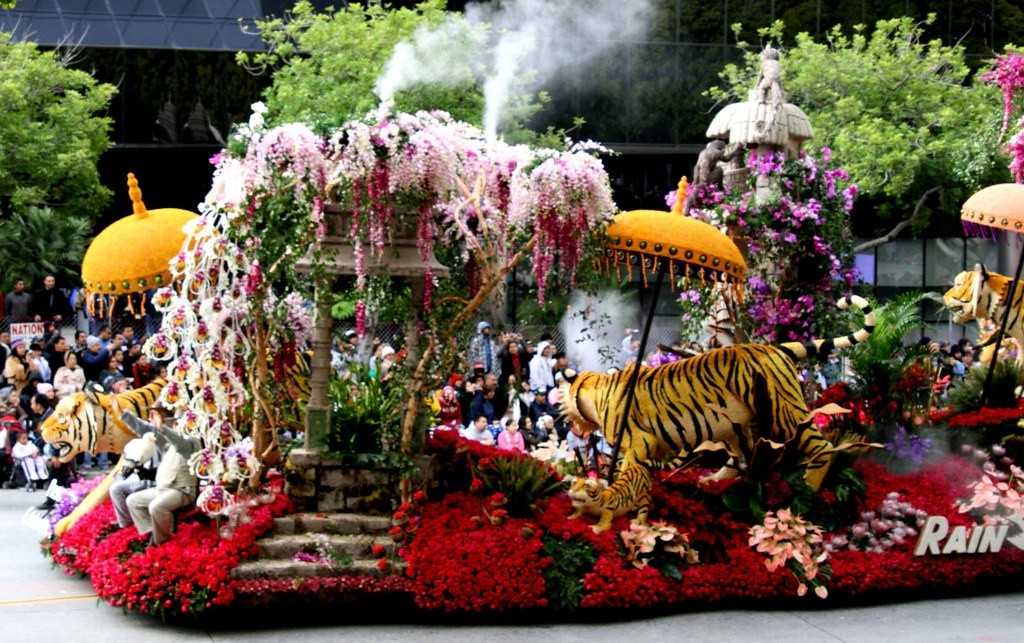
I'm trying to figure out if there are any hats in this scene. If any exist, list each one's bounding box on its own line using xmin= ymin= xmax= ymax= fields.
xmin=535 ymin=387 xmax=546 ymax=394
xmin=473 ymin=361 xmax=484 ymax=369
xmin=37 ymin=383 xmax=54 ymax=395
xmin=145 ymin=399 xmax=174 ymax=417
xmin=44 ymin=321 xmax=53 ymax=331
xmin=0 ymin=385 xmax=14 ymax=404
xmin=32 ymin=336 xmax=44 ymax=344
xmin=86 ymin=335 xmax=100 ymax=349
xmin=382 ymin=346 xmax=395 ymax=360
xmin=11 ymin=336 xmax=25 ymax=349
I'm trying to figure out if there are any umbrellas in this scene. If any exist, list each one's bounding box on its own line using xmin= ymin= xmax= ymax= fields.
xmin=571 ymin=175 xmax=746 ymax=487
xmin=961 ymin=183 xmax=1024 ymax=408
xmin=80 ymin=173 xmax=226 ymax=318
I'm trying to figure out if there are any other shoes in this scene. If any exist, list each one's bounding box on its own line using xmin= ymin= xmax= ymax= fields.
xmin=38 ymin=503 xmax=51 ymax=509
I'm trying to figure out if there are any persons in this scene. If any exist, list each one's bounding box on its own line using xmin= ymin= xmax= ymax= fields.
xmin=917 ymin=336 xmax=982 ymax=402
xmin=27 ymin=275 xmax=76 ymax=334
xmin=29 ymin=392 xmax=73 ymax=510
xmin=328 ymin=320 xmax=723 ymax=475
xmin=5 ymin=278 xmax=31 ymax=322
xmin=0 ymin=321 xmax=168 ymax=491
xmin=125 ymin=407 xmax=203 ymax=546
xmin=71 ymin=277 xmax=164 ymax=337
xmin=797 ymin=348 xmax=857 ymax=399
xmin=107 ymin=392 xmax=177 ymax=528
